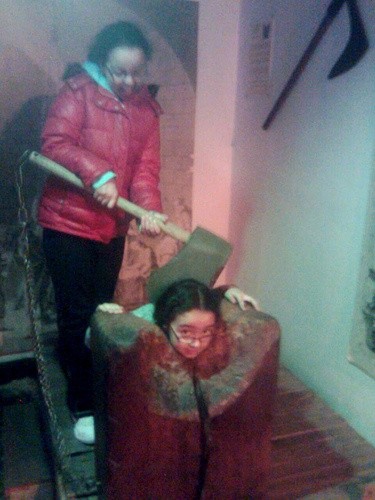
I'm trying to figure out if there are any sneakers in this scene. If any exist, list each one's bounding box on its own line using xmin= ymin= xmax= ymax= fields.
xmin=73 ymin=411 xmax=96 ymax=447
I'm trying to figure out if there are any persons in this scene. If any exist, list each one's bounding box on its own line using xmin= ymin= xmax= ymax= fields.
xmin=36 ymin=22 xmax=170 ymax=445
xmin=83 ymin=279 xmax=262 ymax=361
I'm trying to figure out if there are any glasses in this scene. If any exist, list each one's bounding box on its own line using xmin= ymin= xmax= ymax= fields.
xmin=100 ymin=61 xmax=148 ymax=84
xmin=167 ymin=321 xmax=216 ymax=345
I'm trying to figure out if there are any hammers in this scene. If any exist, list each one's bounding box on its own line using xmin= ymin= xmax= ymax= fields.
xmin=28 ymin=148 xmax=233 ymax=309
